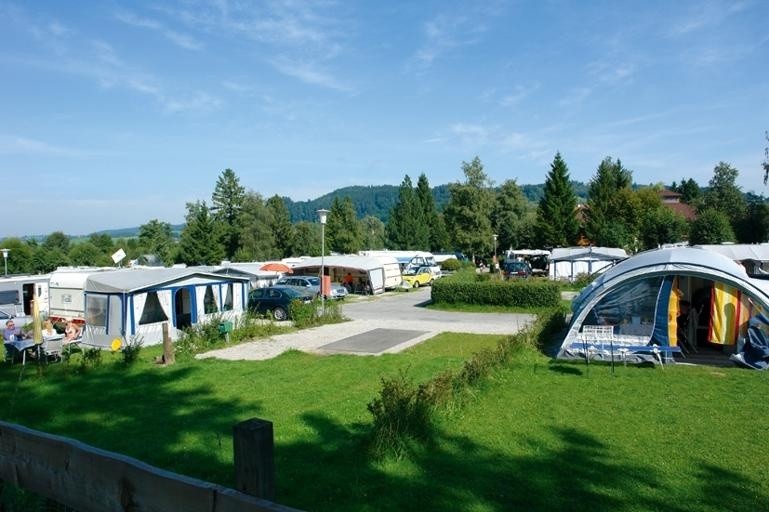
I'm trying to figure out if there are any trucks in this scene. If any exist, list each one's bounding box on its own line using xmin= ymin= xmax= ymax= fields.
xmin=360 ymin=249 xmax=441 ymax=281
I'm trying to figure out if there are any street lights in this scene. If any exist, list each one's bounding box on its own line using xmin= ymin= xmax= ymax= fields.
xmin=315 ymin=208 xmax=331 ymax=312
xmin=491 ymin=235 xmax=500 ymax=264
xmin=0 ymin=248 xmax=12 ymax=277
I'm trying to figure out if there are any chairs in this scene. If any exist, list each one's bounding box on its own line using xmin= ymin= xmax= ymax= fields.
xmin=43 ymin=315 xmax=85 ymax=365
xmin=580 ymin=324 xmax=614 ymax=374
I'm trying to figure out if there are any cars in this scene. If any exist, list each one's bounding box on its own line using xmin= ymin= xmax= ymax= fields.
xmin=401 ymin=266 xmax=435 ymax=288
xmin=0 ymin=310 xmax=32 ymax=330
xmin=503 ymin=260 xmax=530 ymax=280
xmin=248 ymin=275 xmax=348 ymax=321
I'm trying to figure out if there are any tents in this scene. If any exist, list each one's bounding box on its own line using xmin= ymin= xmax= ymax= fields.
xmin=545 ymin=243 xmax=769 ymax=370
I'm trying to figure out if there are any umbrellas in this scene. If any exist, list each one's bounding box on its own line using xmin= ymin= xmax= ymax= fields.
xmin=260 ymin=262 xmax=292 ymax=282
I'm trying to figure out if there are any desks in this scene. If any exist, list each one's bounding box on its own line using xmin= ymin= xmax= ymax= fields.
xmin=2 ymin=334 xmax=65 ymax=366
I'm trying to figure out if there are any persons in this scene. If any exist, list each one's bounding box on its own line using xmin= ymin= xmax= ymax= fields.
xmin=494 ymin=262 xmax=499 ymax=273
xmin=332 ymin=267 xmax=371 ymax=296
xmin=478 ymin=260 xmax=483 ymax=272
xmin=509 ymin=253 xmax=549 ymax=277
xmin=400 ymin=263 xmax=406 ymax=272
xmin=4 ymin=316 xmax=81 ymax=365
